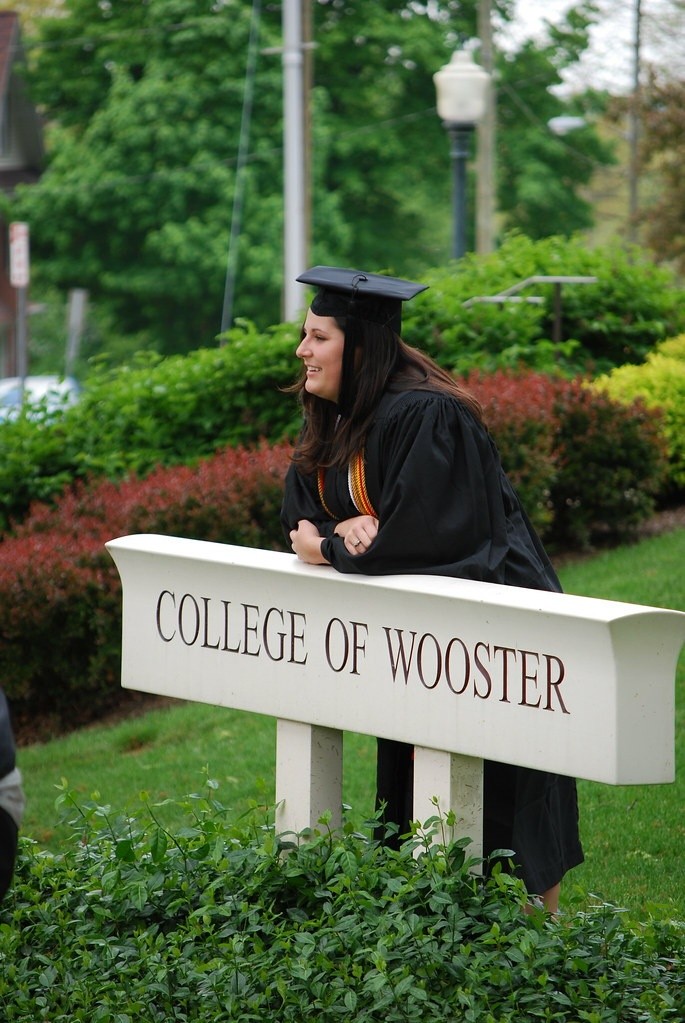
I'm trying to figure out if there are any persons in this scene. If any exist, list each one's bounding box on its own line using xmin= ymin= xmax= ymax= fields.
xmin=280 ymin=266 xmax=584 ymax=918
xmin=0 ymin=691 xmax=26 ymax=896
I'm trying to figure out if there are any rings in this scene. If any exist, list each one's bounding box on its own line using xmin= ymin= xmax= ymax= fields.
xmin=353 ymin=539 xmax=360 ymax=548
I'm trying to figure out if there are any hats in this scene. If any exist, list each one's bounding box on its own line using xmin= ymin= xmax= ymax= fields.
xmin=295 ymin=266 xmax=429 ymax=330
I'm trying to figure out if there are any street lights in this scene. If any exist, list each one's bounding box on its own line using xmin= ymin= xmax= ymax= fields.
xmin=431 ymin=47 xmax=491 ymax=260
